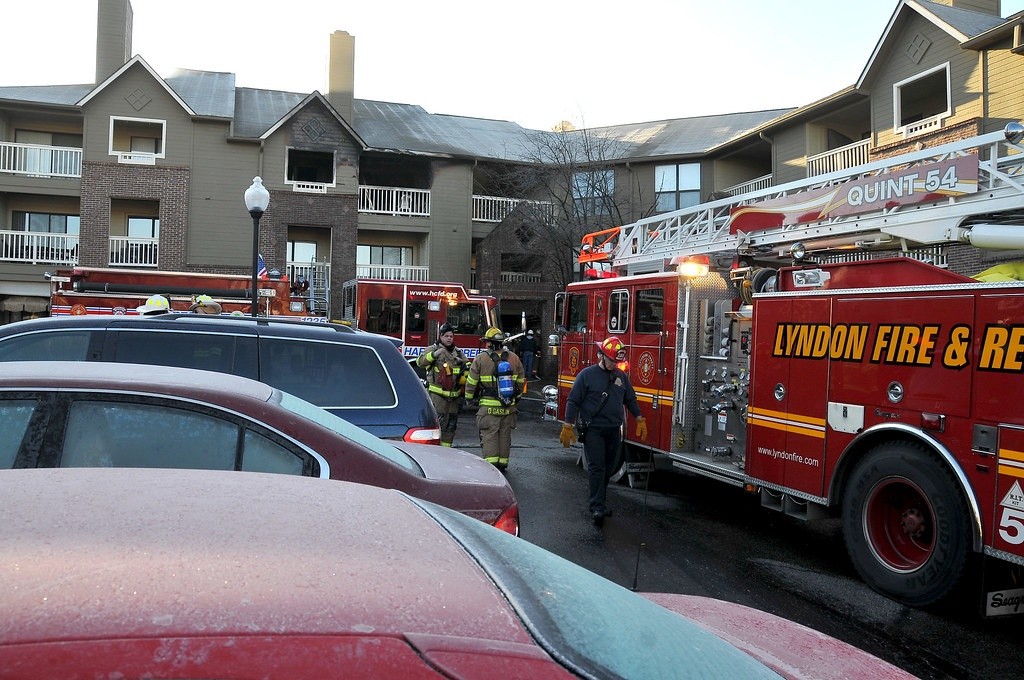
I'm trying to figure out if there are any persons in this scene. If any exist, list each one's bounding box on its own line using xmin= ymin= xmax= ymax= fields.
xmin=188 ymin=295 xmax=221 ymax=314
xmin=413 ymin=311 xmax=421 ymax=319
xmin=136 ymin=293 xmax=171 ymax=316
xmin=464 ymin=327 xmax=527 ymax=468
xmin=290 ymin=274 xmax=309 ymax=295
xmin=519 ymin=328 xmax=541 ymax=378
xmin=559 ymin=337 xmax=648 ymax=520
xmin=416 ymin=322 xmax=472 ymax=447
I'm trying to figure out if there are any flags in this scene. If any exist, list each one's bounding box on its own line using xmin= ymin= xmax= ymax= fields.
xmin=257 ymin=254 xmax=268 ymax=276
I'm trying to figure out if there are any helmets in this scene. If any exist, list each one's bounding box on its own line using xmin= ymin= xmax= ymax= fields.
xmin=478 ymin=327 xmax=504 ymax=341
xmin=439 ymin=322 xmax=455 ymax=336
xmin=188 ymin=294 xmax=222 ymax=313
xmin=136 ymin=294 xmax=173 ymax=313
xmin=594 ymin=337 xmax=628 ymax=361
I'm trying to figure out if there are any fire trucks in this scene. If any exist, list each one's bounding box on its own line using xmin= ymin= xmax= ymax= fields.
xmin=44 ymin=264 xmax=312 ymax=317
xmin=555 ymin=120 xmax=1019 ymax=603
xmin=339 ymin=281 xmax=497 ymax=360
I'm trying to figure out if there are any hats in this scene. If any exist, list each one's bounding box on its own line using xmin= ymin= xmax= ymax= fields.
xmin=528 ymin=330 xmax=533 ymax=334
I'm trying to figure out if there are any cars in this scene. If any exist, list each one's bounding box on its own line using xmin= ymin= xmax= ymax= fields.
xmin=1 ymin=360 xmax=521 ymax=537
xmin=1 ymin=319 xmax=442 ymax=447
xmin=1 ymin=466 xmax=920 ymax=679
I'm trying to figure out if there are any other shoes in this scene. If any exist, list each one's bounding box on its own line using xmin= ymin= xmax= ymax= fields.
xmin=593 ymin=510 xmax=605 ymax=518
xmin=589 ymin=506 xmax=613 ymax=516
xmin=494 ymin=463 xmax=508 ymax=475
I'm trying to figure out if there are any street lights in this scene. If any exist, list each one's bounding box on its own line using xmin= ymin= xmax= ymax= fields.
xmin=242 ymin=177 xmax=270 ymax=314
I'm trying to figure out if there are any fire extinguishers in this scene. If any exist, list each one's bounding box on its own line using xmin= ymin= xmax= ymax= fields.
xmin=497 ymin=360 xmax=515 ymax=406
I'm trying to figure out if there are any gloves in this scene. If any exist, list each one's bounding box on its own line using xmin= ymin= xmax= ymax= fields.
xmin=433 ymin=348 xmax=445 ymax=358
xmin=466 ymin=400 xmax=475 ymax=408
xmin=559 ymin=423 xmax=576 ymax=448
xmin=636 ymin=417 xmax=648 ymax=442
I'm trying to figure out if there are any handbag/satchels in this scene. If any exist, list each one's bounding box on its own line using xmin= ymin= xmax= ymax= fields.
xmin=576 ymin=407 xmax=593 ymax=443
